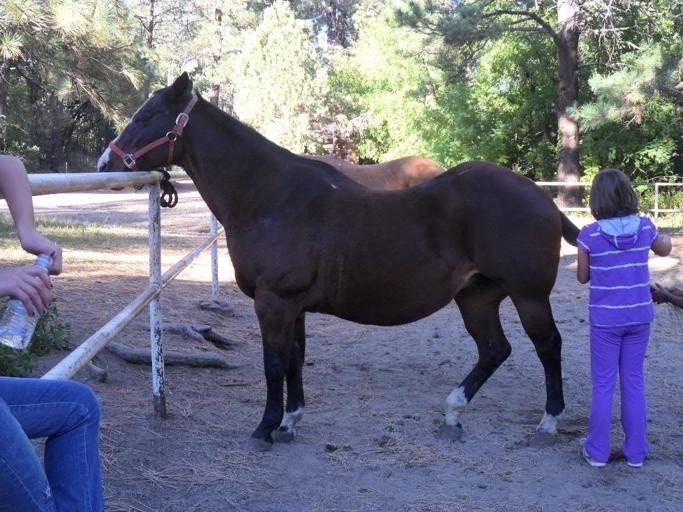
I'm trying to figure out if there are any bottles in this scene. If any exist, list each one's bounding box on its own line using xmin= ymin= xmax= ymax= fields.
xmin=0 ymin=252 xmax=53 ymax=351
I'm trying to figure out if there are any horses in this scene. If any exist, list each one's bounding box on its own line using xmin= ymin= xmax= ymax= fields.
xmin=299 ymin=155 xmax=447 ymax=190
xmin=96 ymin=71 xmax=580 ymax=451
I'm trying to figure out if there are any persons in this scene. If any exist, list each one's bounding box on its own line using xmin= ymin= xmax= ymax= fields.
xmin=0 ymin=153 xmax=103 ymax=512
xmin=577 ymin=169 xmax=672 ymax=468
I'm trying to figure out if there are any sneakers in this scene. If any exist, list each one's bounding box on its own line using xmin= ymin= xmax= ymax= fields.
xmin=579 ymin=438 xmax=604 ymax=467
